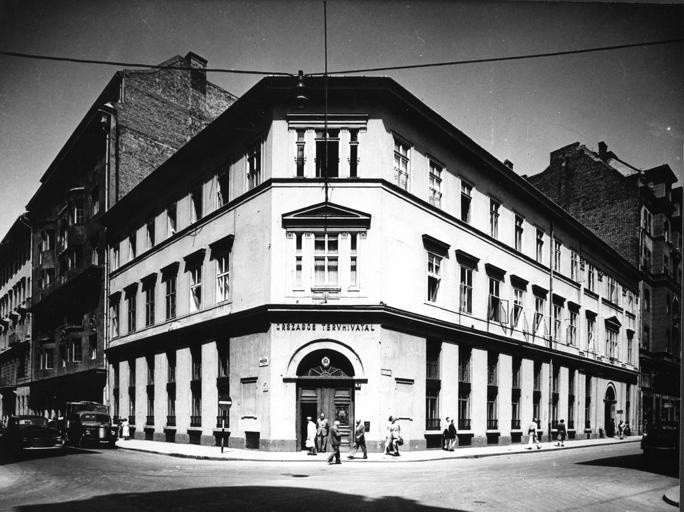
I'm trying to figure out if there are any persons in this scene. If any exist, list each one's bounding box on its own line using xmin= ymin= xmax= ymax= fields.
xmin=557 ymin=420 xmax=566 ymax=446
xmin=443 ymin=417 xmax=450 ymax=450
xmin=607 ymin=418 xmax=629 ymax=439
xmin=448 ymin=420 xmax=458 ymax=451
xmin=305 ymin=413 xmax=403 ymax=464
xmin=527 ymin=418 xmax=541 ymax=449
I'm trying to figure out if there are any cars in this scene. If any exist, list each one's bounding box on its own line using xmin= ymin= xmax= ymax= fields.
xmin=67 ymin=410 xmax=119 ymax=449
xmin=7 ymin=414 xmax=59 ymax=448
xmin=641 ymin=421 xmax=681 ymax=458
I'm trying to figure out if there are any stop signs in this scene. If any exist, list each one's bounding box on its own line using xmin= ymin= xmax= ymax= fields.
xmin=218 ymin=395 xmax=232 ymax=410
